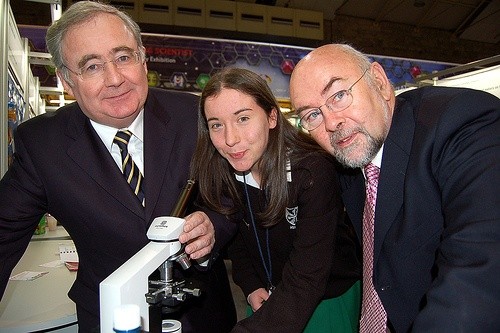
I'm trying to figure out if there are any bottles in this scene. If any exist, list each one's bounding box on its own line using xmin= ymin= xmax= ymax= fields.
xmin=111 ymin=304 xmax=150 ymax=333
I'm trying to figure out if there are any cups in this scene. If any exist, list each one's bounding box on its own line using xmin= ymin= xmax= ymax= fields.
xmin=46 ymin=215 xmax=58 ymax=231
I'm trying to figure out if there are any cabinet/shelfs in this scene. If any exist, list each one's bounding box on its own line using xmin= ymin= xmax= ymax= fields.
xmin=0 ymin=0 xmax=46 ymax=183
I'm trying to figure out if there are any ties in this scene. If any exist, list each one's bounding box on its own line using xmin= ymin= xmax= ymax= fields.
xmin=112 ymin=130 xmax=144 ymax=206
xmin=360 ymin=162 xmax=388 ymax=333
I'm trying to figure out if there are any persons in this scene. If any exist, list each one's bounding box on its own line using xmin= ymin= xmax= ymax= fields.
xmin=290 ymin=44 xmax=500 ymax=333
xmin=189 ymin=68 xmax=362 ymax=333
xmin=0 ymin=0 xmax=244 ymax=333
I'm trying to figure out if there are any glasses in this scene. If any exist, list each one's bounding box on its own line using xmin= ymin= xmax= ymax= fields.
xmin=297 ymin=69 xmax=369 ymax=133
xmin=61 ymin=46 xmax=143 ymax=82
